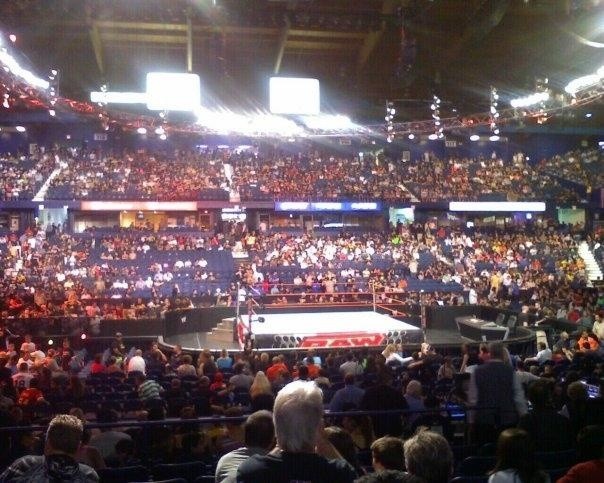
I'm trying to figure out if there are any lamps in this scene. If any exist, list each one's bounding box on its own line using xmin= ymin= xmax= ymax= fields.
xmin=145 ymin=70 xmax=201 ymax=112
xmin=262 ymin=72 xmax=322 ymax=117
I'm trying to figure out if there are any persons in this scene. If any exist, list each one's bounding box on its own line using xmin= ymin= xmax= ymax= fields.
xmin=0 ymin=146 xmax=604 ymax=482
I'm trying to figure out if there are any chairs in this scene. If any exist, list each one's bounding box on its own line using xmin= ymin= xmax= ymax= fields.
xmin=0 ymin=120 xmax=604 ymax=483
